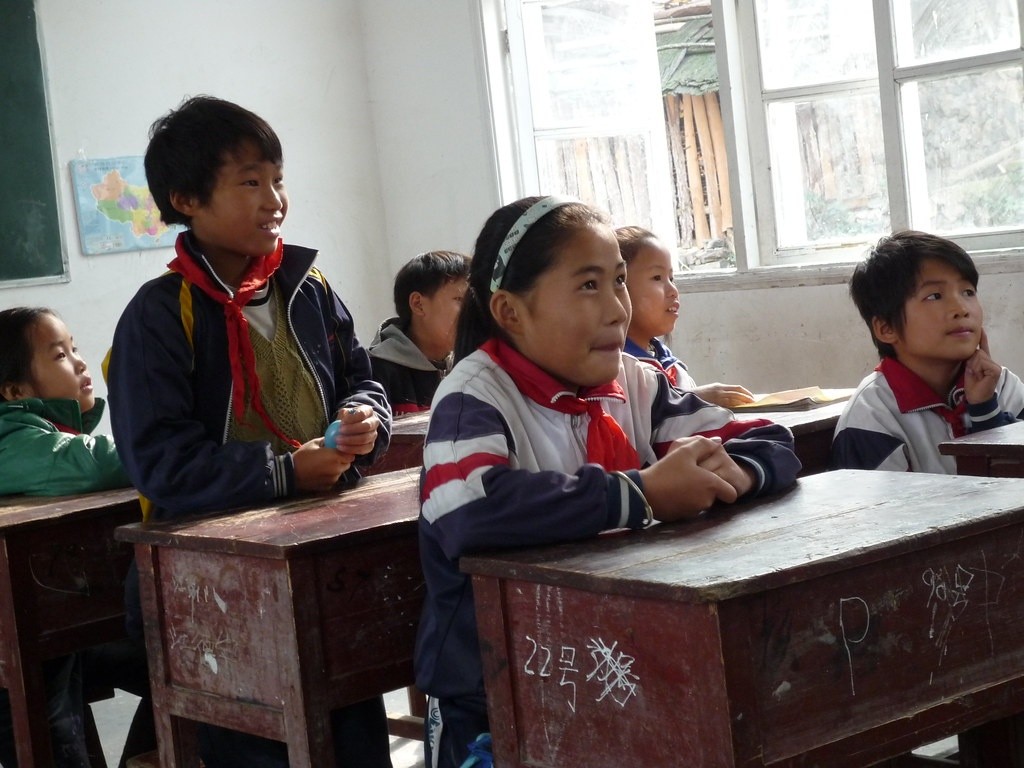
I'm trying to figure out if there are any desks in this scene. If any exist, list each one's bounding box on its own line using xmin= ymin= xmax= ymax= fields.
xmin=718 ymin=387 xmax=862 ymax=478
xmin=459 ymin=466 xmax=1024 ymax=767
xmin=354 ymin=408 xmax=449 ymax=478
xmin=937 ymin=412 xmax=1024 ymax=478
xmin=0 ymin=479 xmax=144 ymax=768
xmin=112 ymin=462 xmax=426 ymax=768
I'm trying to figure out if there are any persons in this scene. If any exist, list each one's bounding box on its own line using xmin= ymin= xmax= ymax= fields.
xmin=0 ymin=306 xmax=142 ymax=768
xmin=809 ymin=228 xmax=1024 ymax=476
xmin=410 ymin=194 xmax=804 ymax=768
xmin=103 ymin=93 xmax=397 ymax=767
xmin=363 ymin=249 xmax=473 ymax=419
xmin=606 ymin=225 xmax=754 ymax=409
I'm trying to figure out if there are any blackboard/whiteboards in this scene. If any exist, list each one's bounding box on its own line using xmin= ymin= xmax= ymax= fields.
xmin=0 ymin=0 xmax=72 ymax=291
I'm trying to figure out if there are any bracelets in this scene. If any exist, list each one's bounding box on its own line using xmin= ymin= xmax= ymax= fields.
xmin=609 ymin=469 xmax=654 ymax=529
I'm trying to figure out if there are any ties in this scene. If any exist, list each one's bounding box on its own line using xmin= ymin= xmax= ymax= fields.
xmin=642 ymin=360 xmax=678 ymax=387
xmin=166 ymin=230 xmax=304 ymax=449
xmin=478 ymin=336 xmax=640 ymax=472
xmin=930 ymin=401 xmax=968 ymax=439
